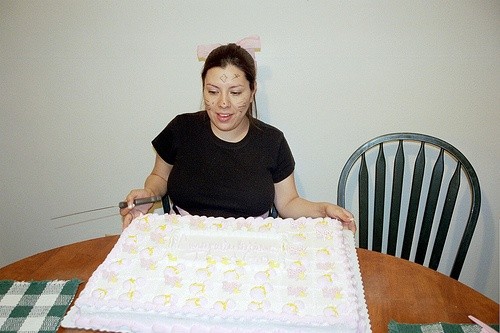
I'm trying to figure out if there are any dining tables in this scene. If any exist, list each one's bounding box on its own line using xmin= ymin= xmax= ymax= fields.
xmin=0 ymin=235 xmax=500 ymax=333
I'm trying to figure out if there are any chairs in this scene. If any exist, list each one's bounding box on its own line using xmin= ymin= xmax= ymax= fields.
xmin=337 ymin=130 xmax=482 ymax=281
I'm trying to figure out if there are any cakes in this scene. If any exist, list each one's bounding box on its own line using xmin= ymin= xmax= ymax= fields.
xmin=59 ymin=213 xmax=371 ymax=333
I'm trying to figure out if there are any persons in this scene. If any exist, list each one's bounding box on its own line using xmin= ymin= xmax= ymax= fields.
xmin=121 ymin=42 xmax=357 ymax=235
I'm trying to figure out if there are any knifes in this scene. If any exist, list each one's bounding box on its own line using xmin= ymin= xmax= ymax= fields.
xmin=51 ymin=194 xmax=163 ymax=221
